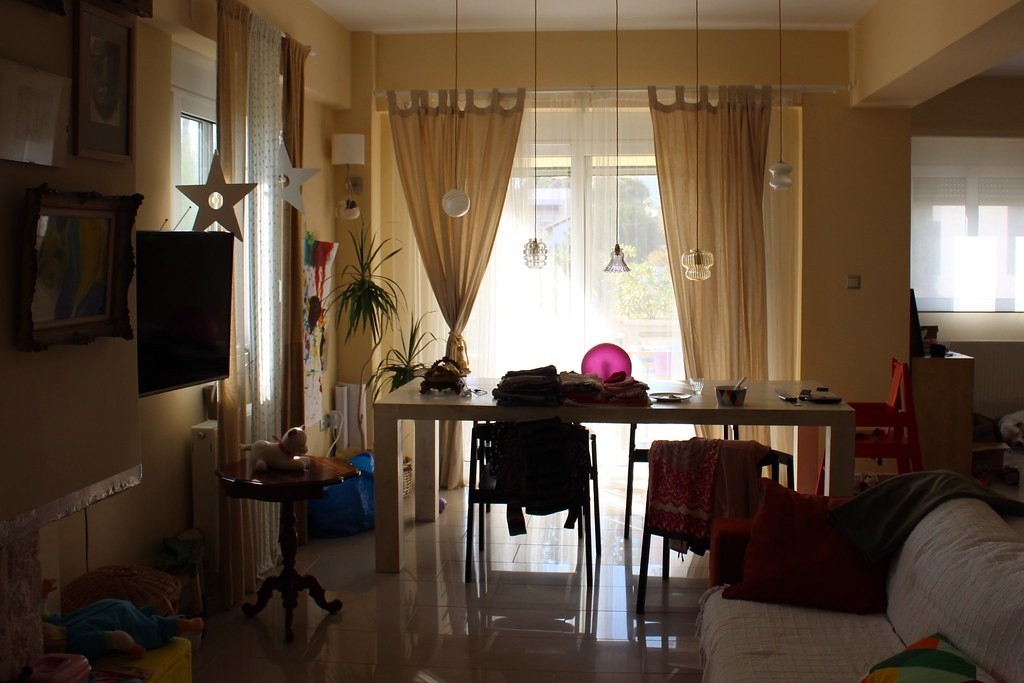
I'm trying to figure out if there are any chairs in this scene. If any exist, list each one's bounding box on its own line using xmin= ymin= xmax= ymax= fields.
xmin=624 ymin=423 xmax=730 ymax=540
xmin=636 ymin=441 xmax=780 ymax=613
xmin=468 ymin=413 xmax=603 ymax=588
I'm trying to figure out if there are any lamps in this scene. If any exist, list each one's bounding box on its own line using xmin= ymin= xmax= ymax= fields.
xmin=439 ymin=0 xmax=470 ymax=219
xmin=681 ymin=0 xmax=716 ymax=282
xmin=329 ymin=132 xmax=366 ymax=196
xmin=522 ymin=1 xmax=550 ymax=271
xmin=603 ymin=0 xmax=632 ymax=274
xmin=768 ymin=0 xmax=793 ymax=191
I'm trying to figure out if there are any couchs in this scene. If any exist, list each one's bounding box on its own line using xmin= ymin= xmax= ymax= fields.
xmin=696 ymin=468 xmax=1024 ymax=683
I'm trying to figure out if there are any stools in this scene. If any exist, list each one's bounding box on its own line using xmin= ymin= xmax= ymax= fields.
xmin=93 ymin=636 xmax=193 ymax=683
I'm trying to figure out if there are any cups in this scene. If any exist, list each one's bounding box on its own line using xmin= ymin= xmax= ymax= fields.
xmin=920 ymin=326 xmax=938 ymax=354
xmin=690 ymin=378 xmax=704 ymax=394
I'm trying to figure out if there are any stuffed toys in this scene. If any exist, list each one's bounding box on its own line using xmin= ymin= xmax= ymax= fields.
xmin=239 ymin=425 xmax=310 ymax=475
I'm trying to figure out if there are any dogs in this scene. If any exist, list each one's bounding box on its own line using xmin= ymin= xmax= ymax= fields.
xmin=239 ymin=424 xmax=312 ymax=476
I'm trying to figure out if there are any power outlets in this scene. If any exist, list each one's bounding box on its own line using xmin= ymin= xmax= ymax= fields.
xmin=321 ymin=420 xmax=329 ymax=430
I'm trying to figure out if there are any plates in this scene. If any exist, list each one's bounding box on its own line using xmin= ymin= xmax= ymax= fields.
xmin=650 ymin=392 xmax=691 ymax=401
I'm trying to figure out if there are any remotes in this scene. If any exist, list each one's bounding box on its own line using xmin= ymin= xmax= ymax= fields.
xmin=775 ymin=388 xmax=844 ymax=404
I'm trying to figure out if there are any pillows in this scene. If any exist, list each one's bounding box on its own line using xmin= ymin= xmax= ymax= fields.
xmin=722 ymin=481 xmax=882 ymax=613
xmin=858 ymin=632 xmax=1000 ymax=683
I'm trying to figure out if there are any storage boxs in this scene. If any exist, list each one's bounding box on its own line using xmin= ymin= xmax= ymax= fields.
xmin=969 ymin=412 xmax=1009 ymax=477
xmin=28 ymin=652 xmax=92 ymax=683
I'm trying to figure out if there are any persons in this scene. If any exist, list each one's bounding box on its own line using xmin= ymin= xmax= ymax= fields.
xmin=44 ymin=598 xmax=203 ymax=655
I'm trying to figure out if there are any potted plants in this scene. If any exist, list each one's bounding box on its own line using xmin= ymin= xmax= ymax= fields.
xmin=332 ymin=219 xmax=433 ymax=499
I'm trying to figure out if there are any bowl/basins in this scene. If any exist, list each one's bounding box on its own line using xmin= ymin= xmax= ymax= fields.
xmin=716 ymin=386 xmax=747 ymax=407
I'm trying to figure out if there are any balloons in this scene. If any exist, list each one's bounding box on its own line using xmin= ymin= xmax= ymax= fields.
xmin=581 ymin=342 xmax=632 ymax=382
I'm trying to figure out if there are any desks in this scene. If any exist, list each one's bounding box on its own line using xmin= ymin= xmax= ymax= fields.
xmin=214 ymin=456 xmax=361 ymax=643
xmin=372 ymin=382 xmax=855 ymax=576
xmin=912 ymin=350 xmax=975 ymax=474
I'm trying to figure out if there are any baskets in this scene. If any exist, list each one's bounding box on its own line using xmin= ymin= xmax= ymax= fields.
xmin=404 ymin=456 xmax=414 ymax=499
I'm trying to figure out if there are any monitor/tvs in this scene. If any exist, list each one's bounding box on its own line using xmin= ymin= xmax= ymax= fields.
xmin=135 ymin=229 xmax=234 ymax=398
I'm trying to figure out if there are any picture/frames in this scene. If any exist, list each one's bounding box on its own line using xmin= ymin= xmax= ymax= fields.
xmin=13 ymin=184 xmax=146 ymax=353
xmin=66 ymin=1 xmax=140 ymax=165
